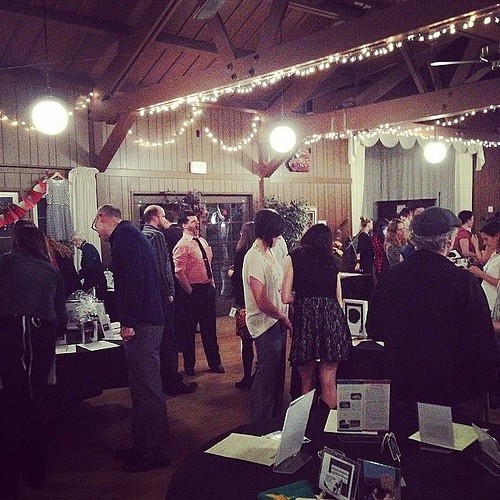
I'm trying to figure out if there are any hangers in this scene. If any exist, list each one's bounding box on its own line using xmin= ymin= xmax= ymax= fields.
xmin=46 ymin=172 xmax=73 ymax=185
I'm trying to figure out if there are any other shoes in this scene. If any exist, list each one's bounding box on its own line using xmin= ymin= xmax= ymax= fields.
xmin=212 ymin=365 xmax=225 ymax=373
xmin=167 ymin=382 xmax=197 ymax=395
xmin=115 ymin=442 xmax=139 ymax=459
xmin=185 ymin=368 xmax=196 ymax=376
xmin=124 ymin=454 xmax=171 ymax=472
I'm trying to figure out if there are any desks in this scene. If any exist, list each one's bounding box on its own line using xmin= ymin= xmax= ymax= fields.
xmin=164 ymin=396 xmax=500 ymax=500
xmin=290 ymin=338 xmax=385 ymax=412
xmin=52 ymin=323 xmax=133 ymax=401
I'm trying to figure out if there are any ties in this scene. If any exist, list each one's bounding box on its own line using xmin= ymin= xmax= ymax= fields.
xmin=193 ymin=237 xmax=212 ymax=279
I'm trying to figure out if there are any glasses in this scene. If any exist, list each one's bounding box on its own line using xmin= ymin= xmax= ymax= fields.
xmin=91 ymin=214 xmax=101 ymax=231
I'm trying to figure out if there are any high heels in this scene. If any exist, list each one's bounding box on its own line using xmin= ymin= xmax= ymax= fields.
xmin=235 ymin=377 xmax=253 ymax=389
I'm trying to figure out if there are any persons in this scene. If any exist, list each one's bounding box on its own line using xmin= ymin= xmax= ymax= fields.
xmin=228 ymin=208 xmax=352 ymax=425
xmin=0 ymin=204 xmax=225 ymax=462
xmin=357 ymin=206 xmax=500 ymax=428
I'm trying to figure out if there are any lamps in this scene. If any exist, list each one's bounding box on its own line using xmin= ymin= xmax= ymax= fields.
xmin=420 ymin=119 xmax=448 ymax=164
xmin=269 ymin=21 xmax=296 ymax=154
xmin=32 ymin=0 xmax=69 ymax=137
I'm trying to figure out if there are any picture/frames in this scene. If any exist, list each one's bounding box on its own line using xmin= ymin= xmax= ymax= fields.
xmin=294 ymin=206 xmax=318 ymax=241
xmin=0 ymin=192 xmax=19 ymax=216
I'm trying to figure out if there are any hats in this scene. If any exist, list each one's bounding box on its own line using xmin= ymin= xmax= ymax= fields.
xmin=410 ymin=206 xmax=462 ymax=237
